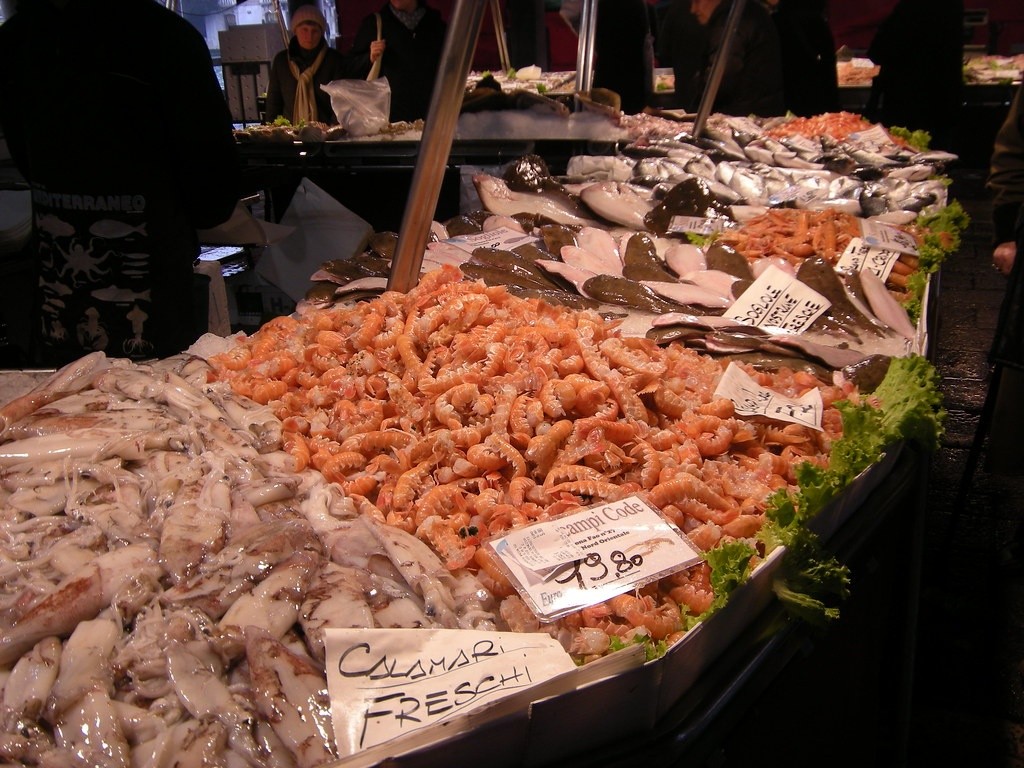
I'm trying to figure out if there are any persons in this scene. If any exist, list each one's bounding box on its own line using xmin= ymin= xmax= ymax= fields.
xmin=536 ymin=0 xmax=596 ymax=167
xmin=591 ymin=1 xmax=843 ymax=114
xmin=982 ymin=81 xmax=1024 ymax=276
xmin=459 ymin=75 xmax=523 ymax=113
xmin=340 ymin=0 xmax=453 ymax=122
xmin=0 ymin=0 xmax=243 ymax=370
xmin=864 ymin=0 xmax=966 ymax=173
xmin=262 ymin=6 xmax=344 ymax=221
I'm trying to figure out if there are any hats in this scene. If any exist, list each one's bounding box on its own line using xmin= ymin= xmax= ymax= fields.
xmin=291 ymin=5 xmax=326 ymax=33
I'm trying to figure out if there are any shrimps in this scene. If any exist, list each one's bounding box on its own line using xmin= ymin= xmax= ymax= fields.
xmin=206 ymin=263 xmax=884 ymax=661
xmin=763 ymin=111 xmax=910 ymax=148
xmin=715 ymin=208 xmax=953 ymax=306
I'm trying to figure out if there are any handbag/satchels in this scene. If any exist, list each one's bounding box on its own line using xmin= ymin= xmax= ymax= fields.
xmin=319 ymin=76 xmax=391 ymax=135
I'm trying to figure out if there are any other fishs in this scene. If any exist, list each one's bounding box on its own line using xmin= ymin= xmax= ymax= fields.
xmin=632 ymin=126 xmax=961 ymax=226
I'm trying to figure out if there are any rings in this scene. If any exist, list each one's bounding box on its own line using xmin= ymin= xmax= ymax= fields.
xmin=991 ymin=264 xmax=995 ymax=272
xmin=995 ymin=267 xmax=1000 ymax=273
